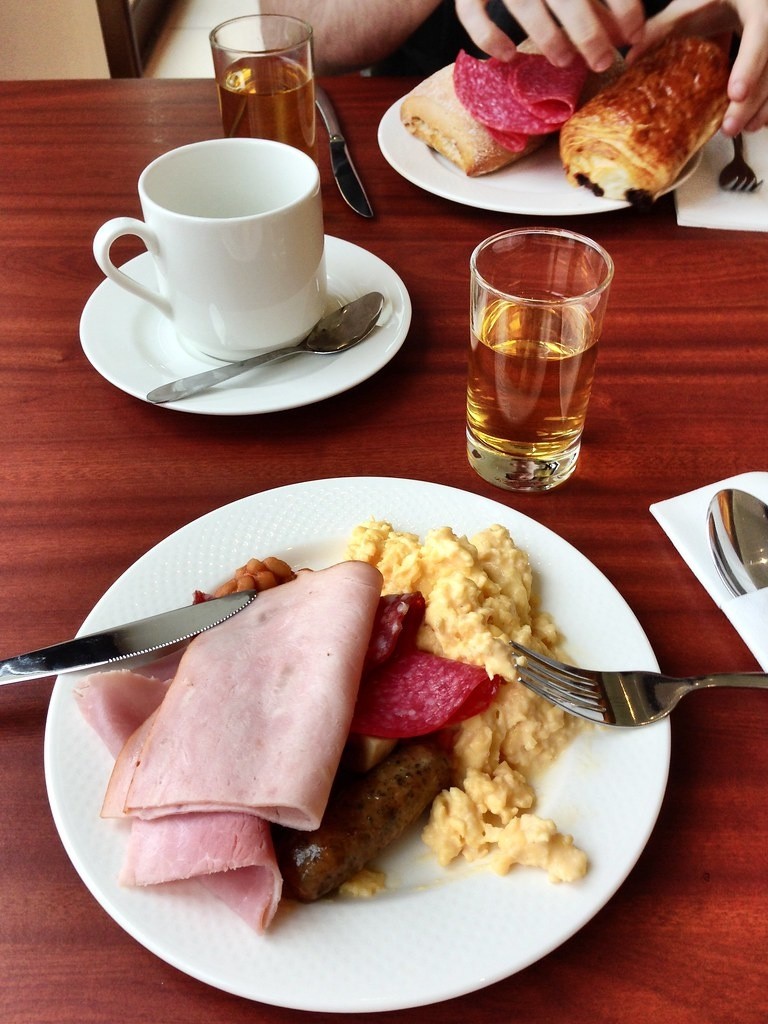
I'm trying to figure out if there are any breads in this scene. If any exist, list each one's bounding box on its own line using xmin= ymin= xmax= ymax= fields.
xmin=399 ymin=27 xmax=628 ymax=176
xmin=561 ymin=34 xmax=734 ymax=205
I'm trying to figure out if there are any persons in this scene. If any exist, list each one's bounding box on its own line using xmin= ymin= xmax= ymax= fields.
xmin=257 ymin=0 xmax=768 ymax=137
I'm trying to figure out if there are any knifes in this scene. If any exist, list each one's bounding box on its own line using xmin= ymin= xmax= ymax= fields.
xmin=0 ymin=592 xmax=259 ymax=685
xmin=314 ymin=87 xmax=375 ymax=219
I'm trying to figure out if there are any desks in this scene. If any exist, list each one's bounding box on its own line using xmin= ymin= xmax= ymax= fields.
xmin=0 ymin=80 xmax=768 ymax=1024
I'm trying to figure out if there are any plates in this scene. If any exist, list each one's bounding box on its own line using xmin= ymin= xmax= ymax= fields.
xmin=376 ymin=93 xmax=707 ymax=217
xmin=80 ymin=236 xmax=412 ymax=416
xmin=43 ymin=473 xmax=672 ymax=1014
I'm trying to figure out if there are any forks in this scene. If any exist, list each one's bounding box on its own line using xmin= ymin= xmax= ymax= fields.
xmin=718 ymin=132 xmax=764 ymax=193
xmin=508 ymin=639 xmax=768 ymax=726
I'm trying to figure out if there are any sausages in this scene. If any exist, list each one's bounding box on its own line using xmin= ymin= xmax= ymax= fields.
xmin=271 ymin=744 xmax=451 ymax=904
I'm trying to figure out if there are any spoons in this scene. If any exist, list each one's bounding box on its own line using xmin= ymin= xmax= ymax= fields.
xmin=706 ymin=488 xmax=768 ymax=597
xmin=146 ymin=290 xmax=386 ymax=403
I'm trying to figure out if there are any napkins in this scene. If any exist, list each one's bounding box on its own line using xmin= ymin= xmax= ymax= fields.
xmin=673 ymin=124 xmax=768 ymax=232
xmin=649 ymin=469 xmax=768 ymax=673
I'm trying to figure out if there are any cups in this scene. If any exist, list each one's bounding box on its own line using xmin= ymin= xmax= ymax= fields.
xmin=92 ymin=137 xmax=328 ymax=363
xmin=208 ymin=13 xmax=319 ymax=169
xmin=463 ymin=225 xmax=615 ymax=491
